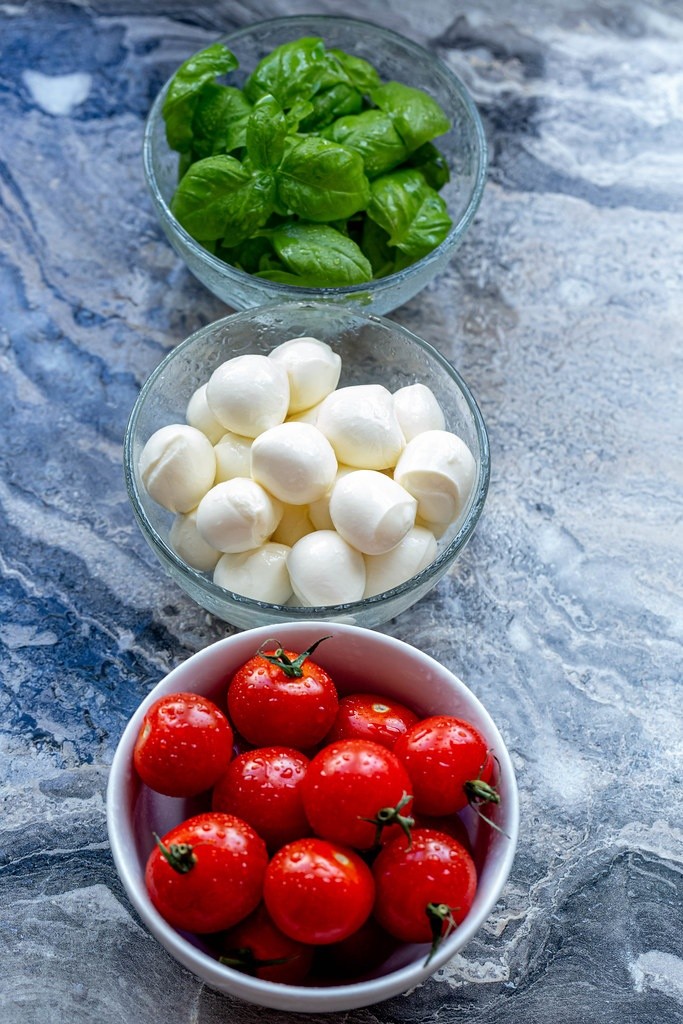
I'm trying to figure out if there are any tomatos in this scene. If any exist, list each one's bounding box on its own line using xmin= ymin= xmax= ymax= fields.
xmin=134 ymin=636 xmax=510 ymax=983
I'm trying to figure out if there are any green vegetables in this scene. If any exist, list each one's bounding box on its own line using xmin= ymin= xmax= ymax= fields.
xmin=161 ymin=36 xmax=453 ymax=292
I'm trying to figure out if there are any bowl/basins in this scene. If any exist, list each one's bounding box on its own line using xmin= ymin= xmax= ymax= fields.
xmin=117 ymin=296 xmax=496 ymax=643
xmin=104 ymin=618 xmax=522 ymax=1019
xmin=142 ymin=15 xmax=487 ymax=333
